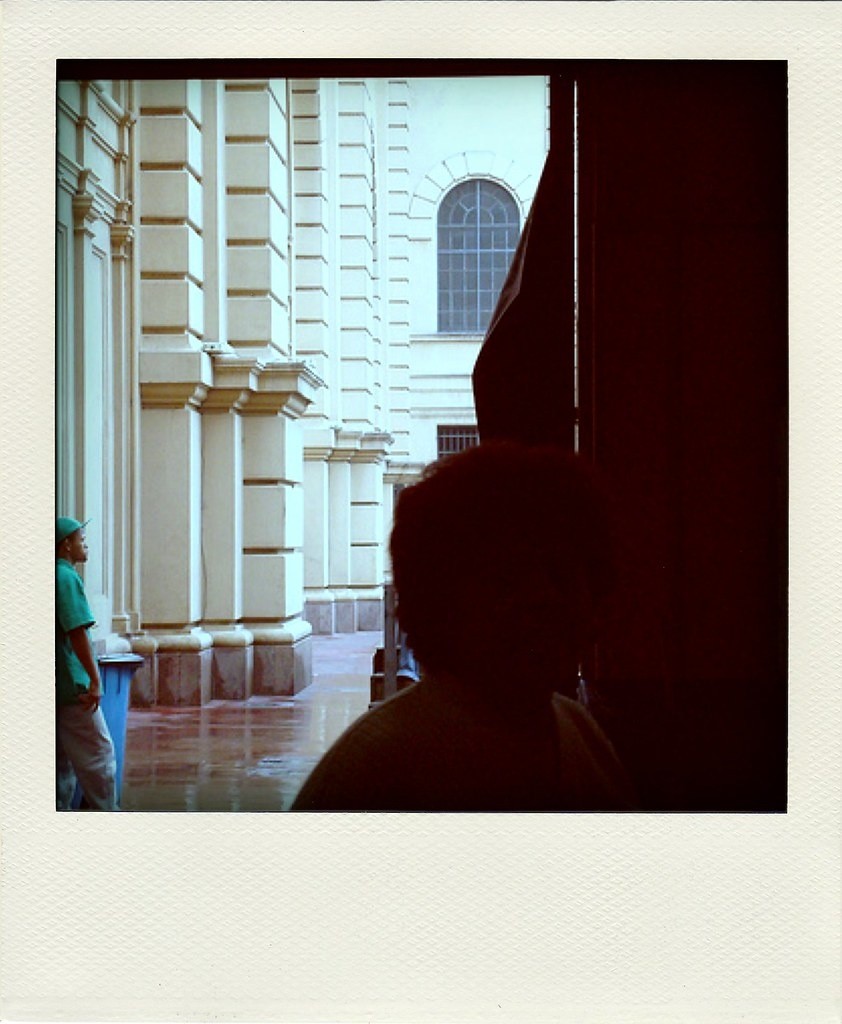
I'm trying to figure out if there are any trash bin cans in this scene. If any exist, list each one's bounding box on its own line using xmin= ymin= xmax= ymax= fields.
xmin=70 ymin=653 xmax=147 ymax=811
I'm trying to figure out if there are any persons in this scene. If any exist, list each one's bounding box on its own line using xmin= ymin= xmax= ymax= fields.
xmin=291 ymin=440 xmax=641 ymax=811
xmin=56 ymin=516 xmax=123 ymax=812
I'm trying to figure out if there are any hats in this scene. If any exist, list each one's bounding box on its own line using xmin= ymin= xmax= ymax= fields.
xmin=56 ymin=518 xmax=90 ymax=544
